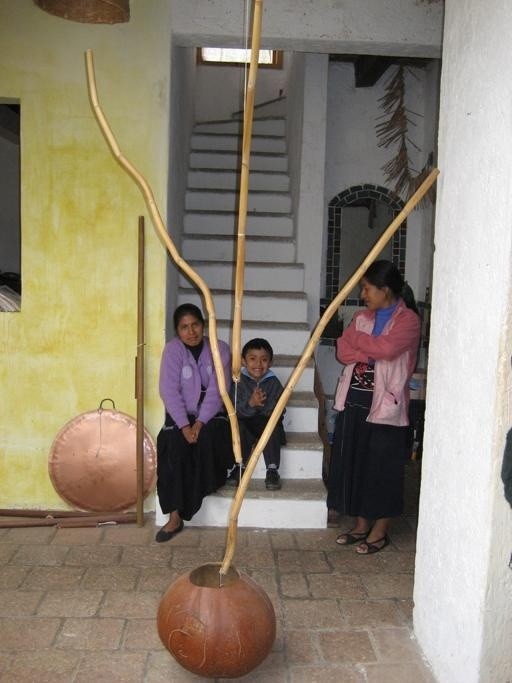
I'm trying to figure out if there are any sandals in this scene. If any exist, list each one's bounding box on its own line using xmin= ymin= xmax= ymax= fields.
xmin=356 ymin=535 xmax=387 ymax=555
xmin=336 ymin=529 xmax=369 ymax=545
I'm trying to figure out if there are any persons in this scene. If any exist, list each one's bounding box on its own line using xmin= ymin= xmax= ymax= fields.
xmin=157 ymin=303 xmax=234 ymax=541
xmin=326 ymin=258 xmax=421 ymax=553
xmin=230 ymin=336 xmax=286 ymax=490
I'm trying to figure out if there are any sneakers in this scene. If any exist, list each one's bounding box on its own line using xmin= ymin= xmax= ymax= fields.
xmin=264 ymin=469 xmax=280 ymax=489
xmin=156 ymin=519 xmax=184 ymax=542
xmin=231 ymin=465 xmax=245 ymax=486
